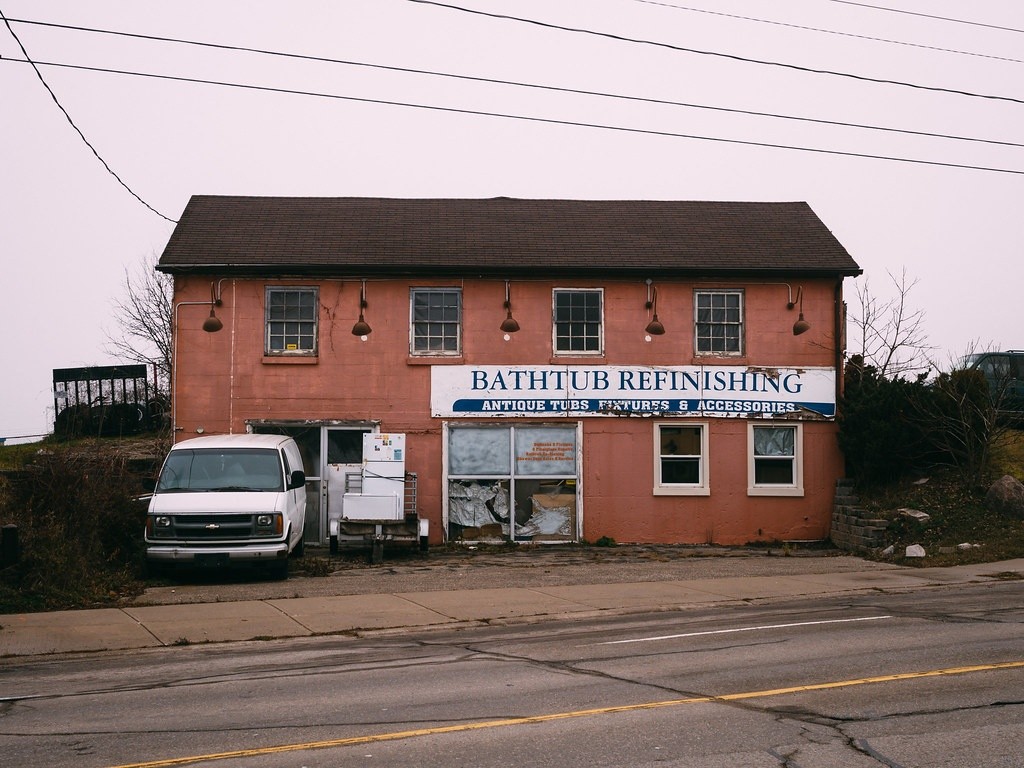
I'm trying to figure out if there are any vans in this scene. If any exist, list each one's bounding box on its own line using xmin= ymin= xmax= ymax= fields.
xmin=145 ymin=433 xmax=306 ymax=583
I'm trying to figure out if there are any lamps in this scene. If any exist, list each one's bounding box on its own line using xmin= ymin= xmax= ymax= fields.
xmin=351 ymin=287 xmax=372 ymax=336
xmin=645 ymin=286 xmax=667 ymax=335
xmin=787 ymin=286 xmax=811 ymax=337
xmin=202 ymin=282 xmax=224 ymax=333
xmin=499 ymin=285 xmax=521 ymax=333
